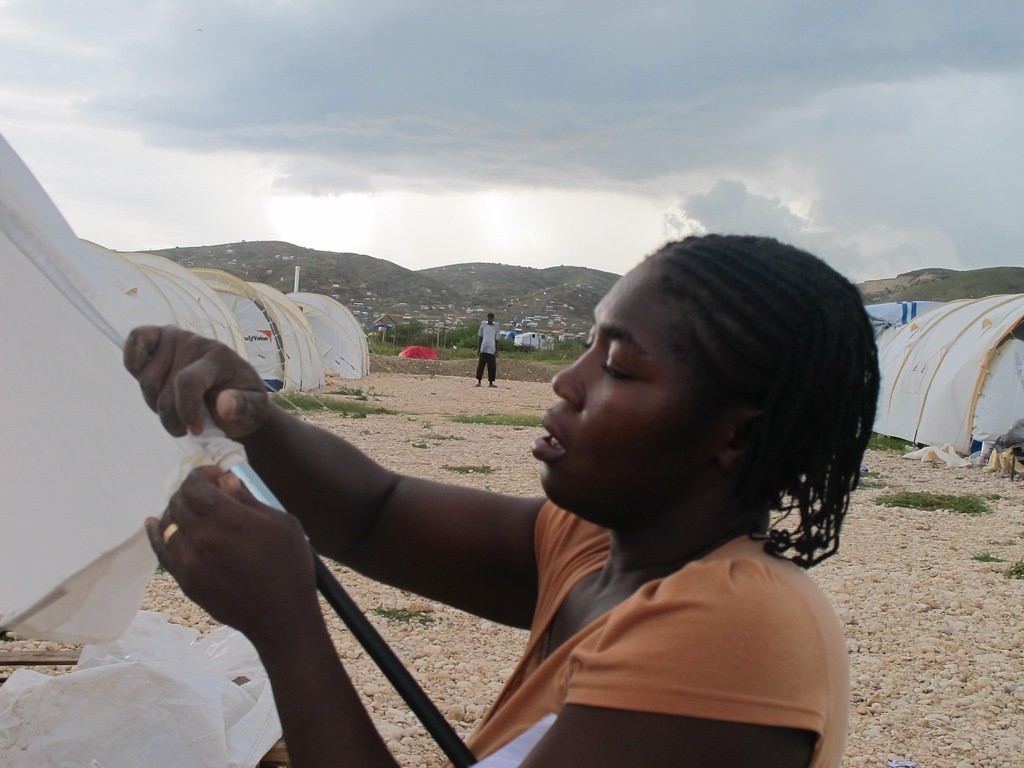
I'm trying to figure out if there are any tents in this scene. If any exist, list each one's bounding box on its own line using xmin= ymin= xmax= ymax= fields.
xmin=0 ymin=134 xmax=370 ymax=625
xmin=864 ymin=294 xmax=1024 ymax=456
xmin=399 ymin=346 xmax=439 ymax=360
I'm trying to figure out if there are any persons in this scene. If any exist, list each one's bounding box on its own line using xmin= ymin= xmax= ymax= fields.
xmin=122 ymin=235 xmax=881 ymax=768
xmin=475 ymin=313 xmax=499 ymax=388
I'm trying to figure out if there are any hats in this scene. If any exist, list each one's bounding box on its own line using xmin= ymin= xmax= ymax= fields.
xmin=487 ymin=313 xmax=494 ymax=318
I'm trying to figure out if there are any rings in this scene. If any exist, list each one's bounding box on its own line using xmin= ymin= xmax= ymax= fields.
xmin=164 ymin=524 xmax=178 ymax=543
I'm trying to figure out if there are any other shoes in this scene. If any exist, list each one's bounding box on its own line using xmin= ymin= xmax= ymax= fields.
xmin=473 ymin=383 xmax=481 ymax=387
xmin=489 ymin=384 xmax=497 ymax=388
